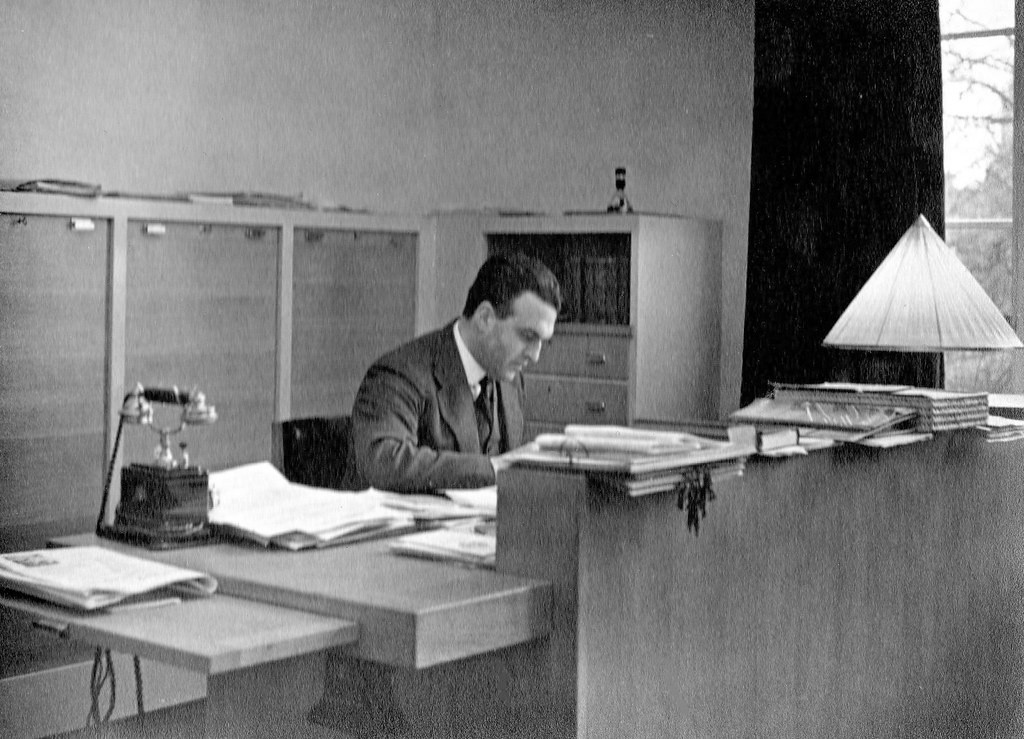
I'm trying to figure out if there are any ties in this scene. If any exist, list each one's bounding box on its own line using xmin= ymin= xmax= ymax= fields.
xmin=476 ymin=376 xmax=493 ymax=430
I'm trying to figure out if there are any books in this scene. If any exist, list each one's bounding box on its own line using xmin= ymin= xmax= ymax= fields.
xmin=17 ymin=179 xmax=297 ymax=206
xmin=0 ymin=383 xmax=1024 ymax=613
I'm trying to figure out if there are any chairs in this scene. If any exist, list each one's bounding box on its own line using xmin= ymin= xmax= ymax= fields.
xmin=271 ymin=415 xmax=351 ymax=490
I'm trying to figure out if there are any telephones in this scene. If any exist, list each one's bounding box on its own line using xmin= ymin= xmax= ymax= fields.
xmin=95 ymin=381 xmax=223 ymax=552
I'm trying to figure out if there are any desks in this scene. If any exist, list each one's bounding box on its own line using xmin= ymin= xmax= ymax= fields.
xmin=0 ymin=415 xmax=1024 ymax=739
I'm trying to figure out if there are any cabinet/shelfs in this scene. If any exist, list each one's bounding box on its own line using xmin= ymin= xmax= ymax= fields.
xmin=480 ymin=210 xmax=724 ymax=449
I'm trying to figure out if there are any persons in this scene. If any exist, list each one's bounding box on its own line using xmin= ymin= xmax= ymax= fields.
xmin=341 ymin=250 xmax=564 ymax=492
